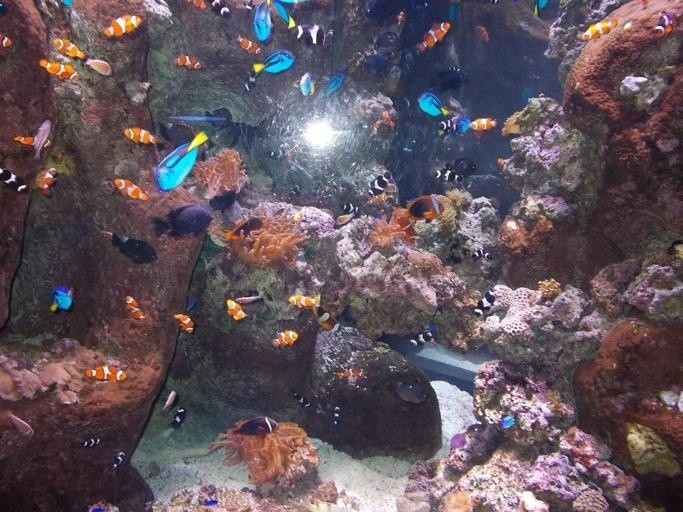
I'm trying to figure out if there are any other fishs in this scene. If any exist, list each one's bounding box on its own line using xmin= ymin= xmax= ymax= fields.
xmin=0 ymin=1 xmax=497 ymax=386
